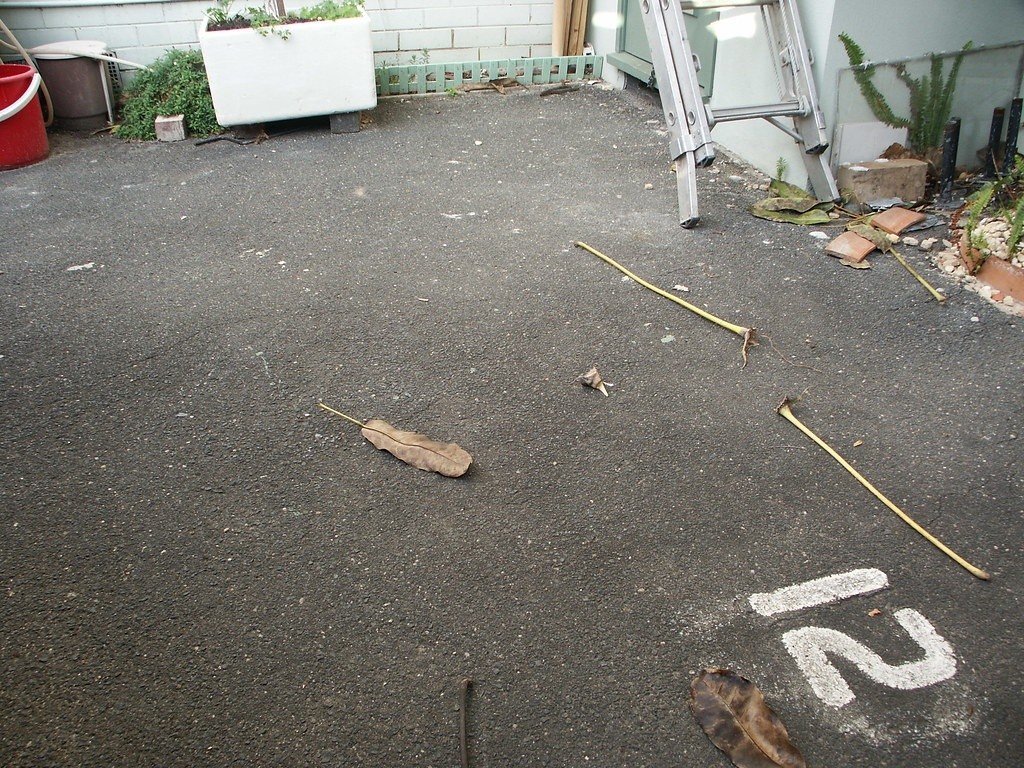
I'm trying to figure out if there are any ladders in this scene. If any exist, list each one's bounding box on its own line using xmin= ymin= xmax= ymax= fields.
xmin=636 ymin=0 xmax=844 ymax=229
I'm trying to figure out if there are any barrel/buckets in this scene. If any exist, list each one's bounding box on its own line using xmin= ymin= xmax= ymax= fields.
xmin=0 ymin=64 xmax=49 ymax=171
xmin=31 ymin=41 xmax=115 ymax=130
